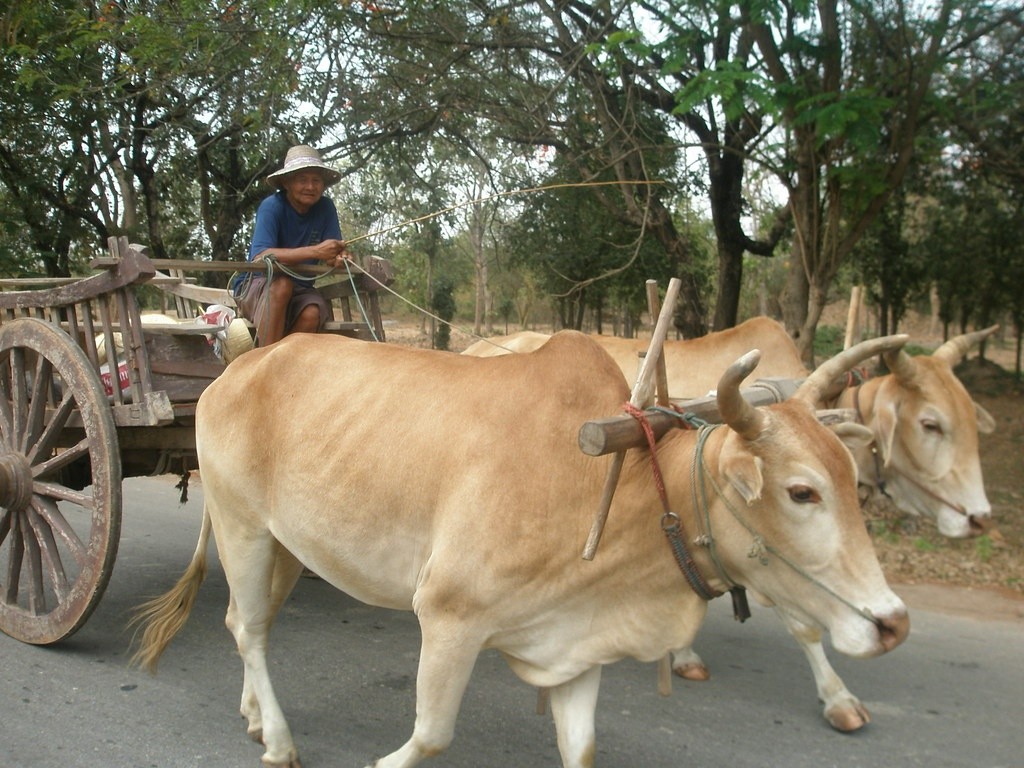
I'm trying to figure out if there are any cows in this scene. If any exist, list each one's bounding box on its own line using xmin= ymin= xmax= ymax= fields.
xmin=129 ymin=313 xmax=1004 ymax=768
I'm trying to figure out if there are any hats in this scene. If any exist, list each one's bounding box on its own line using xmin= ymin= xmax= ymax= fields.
xmin=267 ymin=144 xmax=341 ymax=192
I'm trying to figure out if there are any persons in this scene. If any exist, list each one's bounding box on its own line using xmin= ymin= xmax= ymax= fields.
xmin=234 ymin=146 xmax=354 ymax=347
